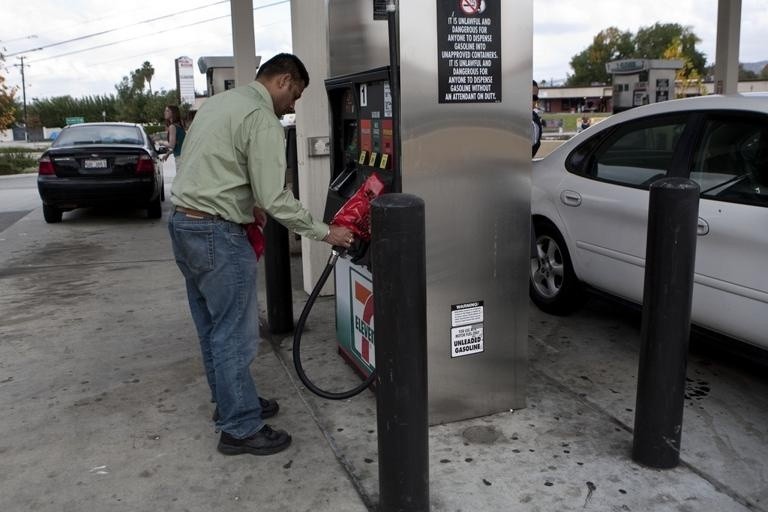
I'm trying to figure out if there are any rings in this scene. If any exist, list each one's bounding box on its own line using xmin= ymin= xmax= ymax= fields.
xmin=348 ymin=239 xmax=352 ymax=244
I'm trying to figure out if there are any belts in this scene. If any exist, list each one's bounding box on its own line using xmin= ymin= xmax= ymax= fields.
xmin=175 ymin=206 xmax=208 ymax=217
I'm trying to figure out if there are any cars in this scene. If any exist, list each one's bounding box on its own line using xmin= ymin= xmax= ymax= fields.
xmin=530 ymin=92 xmax=768 ymax=351
xmin=37 ymin=122 xmax=168 ymax=223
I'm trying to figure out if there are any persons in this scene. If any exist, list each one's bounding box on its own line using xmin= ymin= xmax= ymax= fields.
xmin=532 ymin=80 xmax=542 ymax=158
xmin=168 ymin=53 xmax=358 ymax=455
xmin=157 ymin=105 xmax=186 ymax=172
xmin=581 ymin=116 xmax=590 ymax=129
xmin=558 ymin=118 xmax=563 ymax=134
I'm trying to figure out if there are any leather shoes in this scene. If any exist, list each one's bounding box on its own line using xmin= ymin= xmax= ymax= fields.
xmin=212 ymin=396 xmax=278 ymax=422
xmin=217 ymin=424 xmax=291 ymax=456
